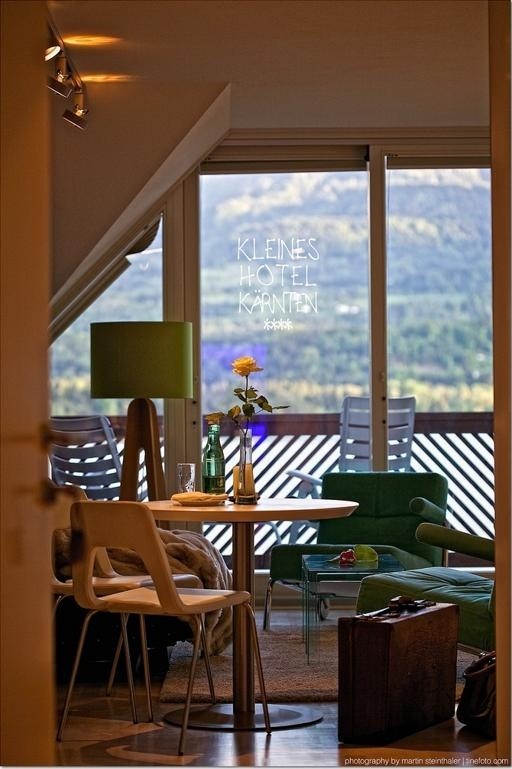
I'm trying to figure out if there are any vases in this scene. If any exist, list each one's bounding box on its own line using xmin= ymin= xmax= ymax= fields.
xmin=233 ymin=427 xmax=256 ymax=506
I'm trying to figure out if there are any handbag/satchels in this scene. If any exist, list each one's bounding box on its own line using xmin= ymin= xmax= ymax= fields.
xmin=456 ymin=651 xmax=496 ymax=729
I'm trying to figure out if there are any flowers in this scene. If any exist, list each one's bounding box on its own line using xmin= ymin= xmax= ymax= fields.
xmin=201 ymin=351 xmax=293 ymax=430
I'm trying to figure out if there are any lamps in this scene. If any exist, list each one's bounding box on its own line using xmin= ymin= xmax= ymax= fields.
xmin=43 ymin=34 xmax=89 ymax=136
xmin=87 ymin=317 xmax=194 ymax=533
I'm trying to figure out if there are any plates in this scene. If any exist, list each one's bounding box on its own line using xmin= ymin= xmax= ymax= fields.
xmin=169 ymin=496 xmax=226 ymax=509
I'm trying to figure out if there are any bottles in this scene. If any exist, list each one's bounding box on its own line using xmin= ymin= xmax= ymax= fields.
xmin=200 ymin=425 xmax=226 ymax=492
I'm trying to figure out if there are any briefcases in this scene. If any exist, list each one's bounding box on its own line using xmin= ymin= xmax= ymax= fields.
xmin=338 ymin=594 xmax=458 ymax=743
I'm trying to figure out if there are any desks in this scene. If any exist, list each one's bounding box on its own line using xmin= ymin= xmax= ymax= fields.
xmin=298 ymin=551 xmax=408 ymax=665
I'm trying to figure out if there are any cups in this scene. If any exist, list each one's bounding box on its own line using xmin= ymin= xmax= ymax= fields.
xmin=176 ymin=461 xmax=196 ymax=494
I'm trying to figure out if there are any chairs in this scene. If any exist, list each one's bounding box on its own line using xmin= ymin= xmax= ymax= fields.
xmin=261 ymin=471 xmax=449 ymax=634
xmin=49 ymin=476 xmax=218 ymax=712
xmin=286 ymin=395 xmax=419 ymax=547
xmin=56 ymin=499 xmax=276 ymax=756
xmin=48 ymin=413 xmax=285 ymax=562
xmin=352 ymin=522 xmax=496 ymax=654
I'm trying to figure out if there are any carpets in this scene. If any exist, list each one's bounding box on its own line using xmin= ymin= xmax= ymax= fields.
xmin=153 ymin=620 xmax=473 ymax=705
